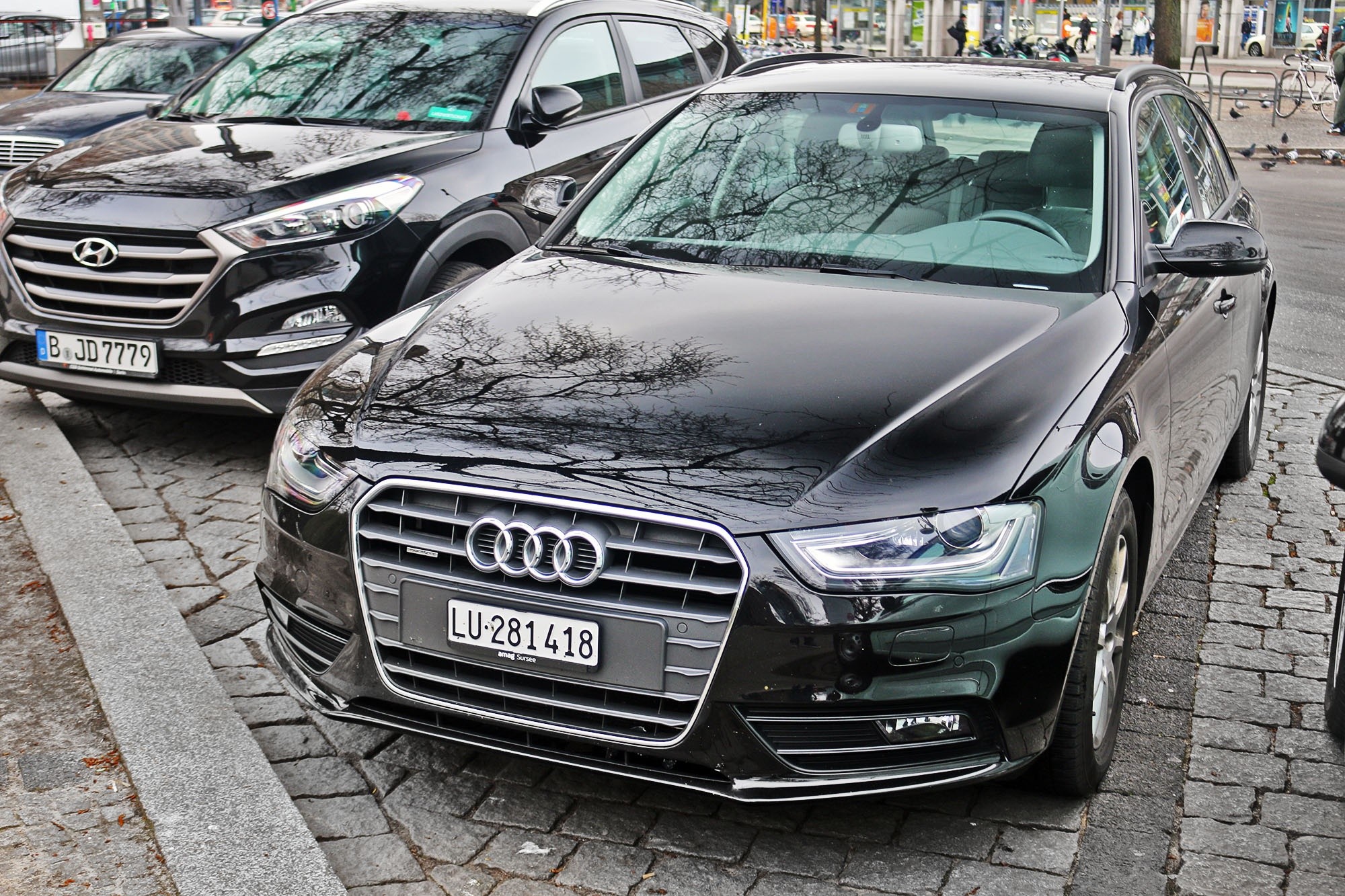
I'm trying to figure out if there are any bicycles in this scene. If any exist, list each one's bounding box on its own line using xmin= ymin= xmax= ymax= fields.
xmin=1273 ymin=53 xmax=1340 ymax=125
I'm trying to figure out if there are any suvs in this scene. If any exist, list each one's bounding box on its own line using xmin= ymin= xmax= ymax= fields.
xmin=0 ymin=1 xmax=758 ymax=431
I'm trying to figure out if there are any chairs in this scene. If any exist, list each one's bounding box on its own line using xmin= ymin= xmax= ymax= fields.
xmin=159 ymin=62 xmax=190 ymax=85
xmin=756 ymin=118 xmax=1093 ymax=251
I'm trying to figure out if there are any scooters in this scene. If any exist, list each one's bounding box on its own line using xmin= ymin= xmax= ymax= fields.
xmin=964 ymin=21 xmax=1007 ymax=60
xmin=1004 ymin=30 xmax=1036 ymax=58
xmin=1043 ymin=34 xmax=1081 ymax=63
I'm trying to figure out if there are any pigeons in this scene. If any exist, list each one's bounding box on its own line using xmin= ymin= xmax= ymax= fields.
xmin=1230 ymin=88 xmax=1345 ymax=171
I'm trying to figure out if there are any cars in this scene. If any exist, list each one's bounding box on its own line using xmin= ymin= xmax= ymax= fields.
xmin=1040 ymin=16 xmax=1110 ymax=49
xmin=1245 ymin=21 xmax=1329 ymax=58
xmin=748 ymin=12 xmax=764 ymax=37
xmin=0 ymin=10 xmax=80 ymax=83
xmin=99 ymin=7 xmax=306 ymax=42
xmin=1007 ymin=16 xmax=1035 ymax=41
xmin=735 ymin=12 xmax=847 ymax=61
xmin=0 ymin=24 xmax=271 ymax=185
xmin=240 ymin=49 xmax=1280 ymax=799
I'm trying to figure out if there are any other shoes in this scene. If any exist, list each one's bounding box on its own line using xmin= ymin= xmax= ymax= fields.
xmin=1327 ymin=127 xmax=1342 ymax=136
xmin=1130 ymin=53 xmax=1135 ymax=55
xmin=1315 ymin=50 xmax=1321 ymax=56
xmin=1115 ymin=52 xmax=1120 ymax=55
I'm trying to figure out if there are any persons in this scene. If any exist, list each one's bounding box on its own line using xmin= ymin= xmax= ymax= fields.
xmin=1079 ymin=12 xmax=1093 ymax=52
xmin=1109 ymin=11 xmax=1124 ymax=55
xmin=1129 ymin=11 xmax=1154 ymax=57
xmin=1241 ymin=15 xmax=1252 ymax=50
xmin=954 ymin=13 xmax=968 ymax=56
xmin=1062 ymin=8 xmax=1072 ymax=43
xmin=1315 ymin=26 xmax=1329 ymax=60
xmin=1326 ymin=42 xmax=1345 ymax=134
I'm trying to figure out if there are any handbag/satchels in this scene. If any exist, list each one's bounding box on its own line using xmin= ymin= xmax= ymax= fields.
xmin=1111 ymin=37 xmax=1121 ymax=48
xmin=948 ymin=27 xmax=960 ymax=38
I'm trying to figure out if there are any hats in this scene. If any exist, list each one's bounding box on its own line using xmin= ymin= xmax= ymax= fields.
xmin=1323 ymin=26 xmax=1328 ymax=29
